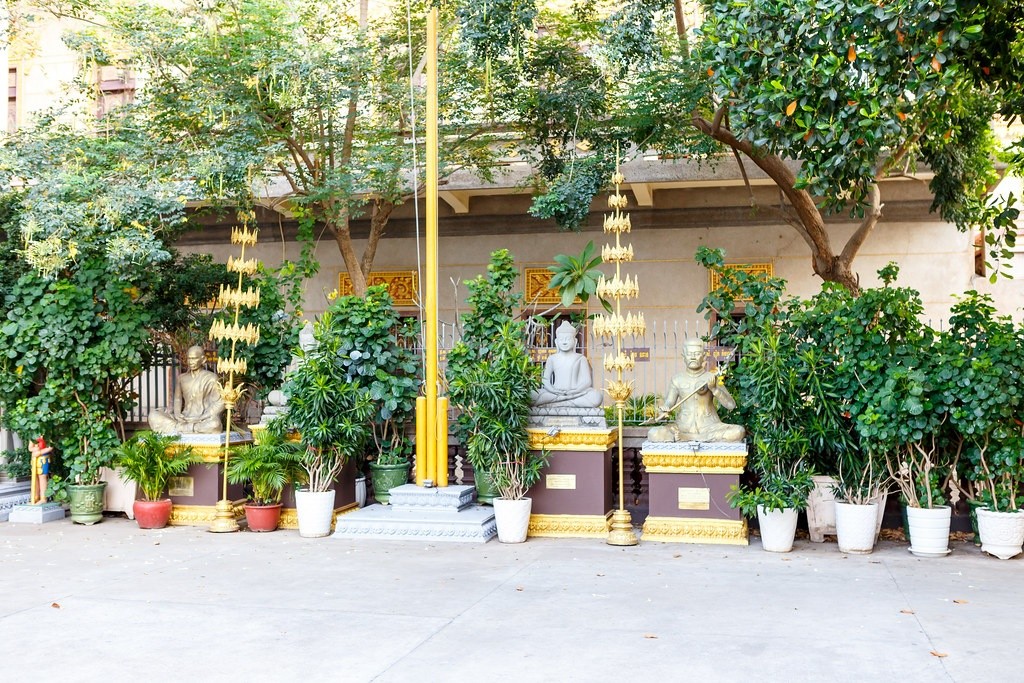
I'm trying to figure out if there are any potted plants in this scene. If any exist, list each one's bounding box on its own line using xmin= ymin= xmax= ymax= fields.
xmin=115 ymin=429 xmax=194 ymax=529
xmin=464 ymin=432 xmax=552 ymax=542
xmin=693 ymin=245 xmax=1024 ymax=561
xmin=220 ymin=425 xmax=303 ymax=533
xmin=352 ymin=443 xmax=368 ymax=508
xmin=340 ymin=287 xmax=420 ymax=505
xmin=281 ymin=315 xmax=370 ymax=537
xmin=67 ymin=411 xmax=117 ymax=525
xmin=450 ymin=244 xmax=535 ymax=505
